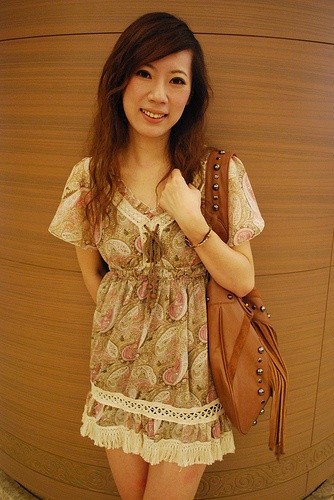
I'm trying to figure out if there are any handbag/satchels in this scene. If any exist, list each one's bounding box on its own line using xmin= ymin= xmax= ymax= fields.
xmin=202 ymin=148 xmax=287 ymax=462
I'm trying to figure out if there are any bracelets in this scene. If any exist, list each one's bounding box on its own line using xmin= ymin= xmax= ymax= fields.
xmin=188 ymin=228 xmax=214 ymax=250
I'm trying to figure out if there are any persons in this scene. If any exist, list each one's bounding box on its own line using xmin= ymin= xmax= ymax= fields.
xmin=49 ymin=10 xmax=288 ymax=499
